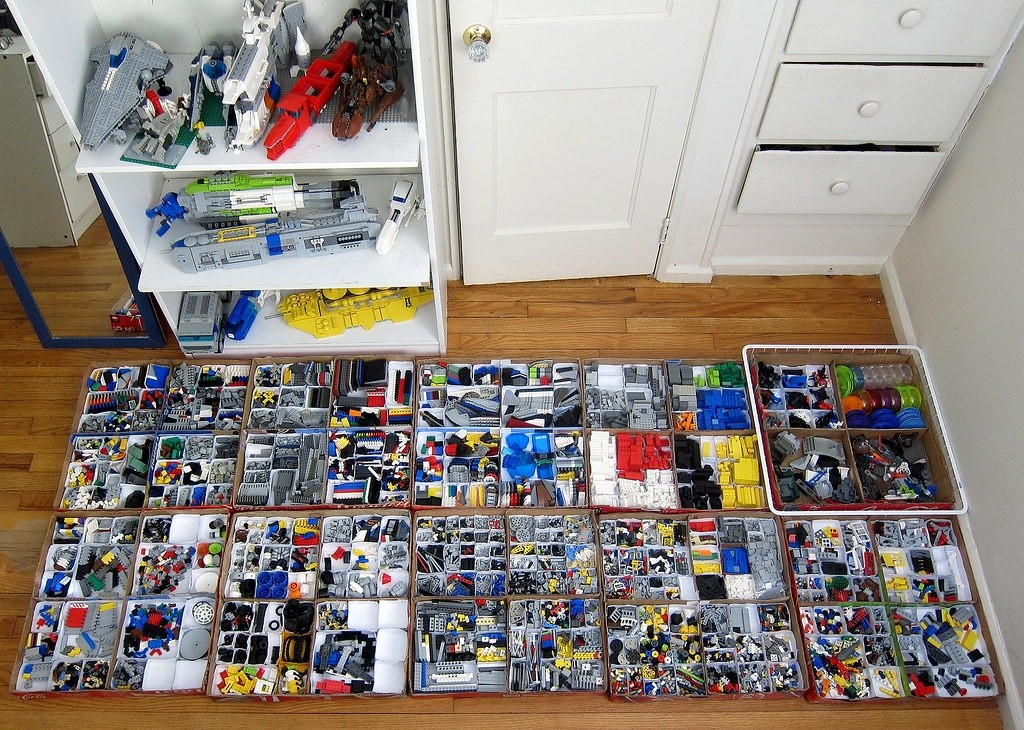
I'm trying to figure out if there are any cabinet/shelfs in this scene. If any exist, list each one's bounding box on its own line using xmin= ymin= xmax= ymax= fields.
xmin=0 ymin=36 xmax=103 ymax=249
xmin=709 ymin=0 xmax=1024 ymax=277
xmin=6 ymin=0 xmax=450 ymax=357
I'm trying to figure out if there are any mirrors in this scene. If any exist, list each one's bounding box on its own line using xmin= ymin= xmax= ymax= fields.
xmin=0 ymin=0 xmax=168 ymax=351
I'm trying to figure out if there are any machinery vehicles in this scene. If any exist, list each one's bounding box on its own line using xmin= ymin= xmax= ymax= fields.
xmin=77 ymin=0 xmax=436 ymax=339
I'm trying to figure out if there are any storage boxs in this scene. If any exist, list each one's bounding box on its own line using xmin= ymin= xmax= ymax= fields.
xmin=410 ymin=508 xmax=609 ymax=696
xmin=415 ymin=355 xmax=591 ymax=509
xmin=206 ymin=507 xmax=411 ymax=701
xmin=582 ymin=357 xmax=771 ymax=512
xmin=50 ymin=359 xmax=253 ymax=513
xmin=232 ymin=356 xmax=416 ymax=509
xmin=108 ymin=286 xmax=145 ymax=332
xmin=7 ymin=507 xmax=230 ymax=697
xmin=781 ymin=513 xmax=1005 ymax=703
xmin=751 ymin=351 xmax=957 ymax=510
xmin=596 ymin=511 xmax=811 ymax=703
xmin=742 ymin=342 xmax=969 ymax=515
